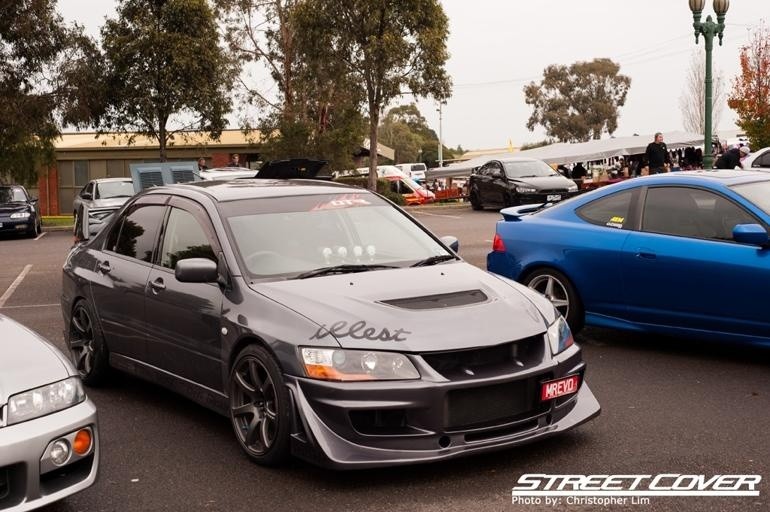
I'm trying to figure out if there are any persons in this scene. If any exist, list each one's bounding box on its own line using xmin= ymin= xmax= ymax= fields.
xmin=715 ymin=147 xmax=750 ymax=169
xmin=198 ymin=157 xmax=208 ymax=170
xmin=613 ymin=158 xmax=624 ymax=171
xmin=645 ymin=133 xmax=674 ymax=175
xmin=227 ymin=154 xmax=242 ymax=166
xmin=572 ymin=161 xmax=588 ymax=178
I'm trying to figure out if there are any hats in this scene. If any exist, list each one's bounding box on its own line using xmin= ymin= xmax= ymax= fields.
xmin=740 ymin=146 xmax=751 ymax=156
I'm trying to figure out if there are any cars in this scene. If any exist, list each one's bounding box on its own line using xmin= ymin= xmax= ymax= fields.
xmin=1 ymin=147 xmax=770 ymax=511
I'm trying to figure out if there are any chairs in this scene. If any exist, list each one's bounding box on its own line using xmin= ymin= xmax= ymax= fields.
xmin=660 ymin=194 xmax=735 ymax=236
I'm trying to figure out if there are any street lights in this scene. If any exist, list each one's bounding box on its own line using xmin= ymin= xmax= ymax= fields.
xmin=688 ymin=0 xmax=730 ymax=170
xmin=436 ymin=99 xmax=443 ymax=167
